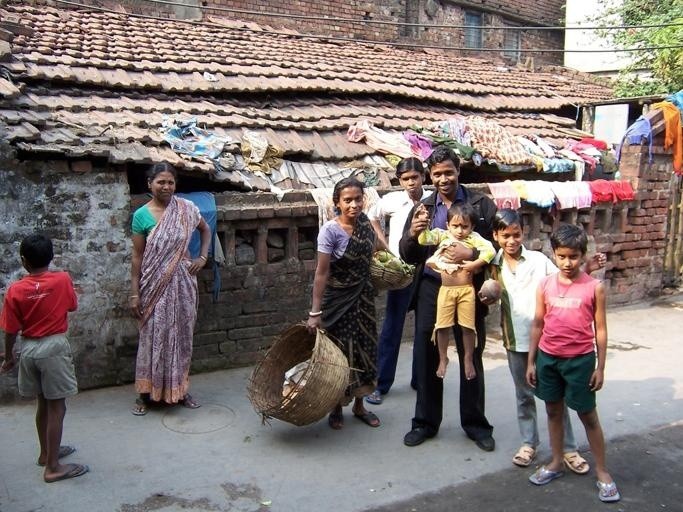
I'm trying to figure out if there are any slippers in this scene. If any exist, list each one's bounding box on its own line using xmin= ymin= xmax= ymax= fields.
xmin=178 ymin=391 xmax=201 ymax=411
xmin=366 ymin=389 xmax=382 ymax=405
xmin=44 ymin=462 xmax=88 ymax=484
xmin=329 ymin=411 xmax=346 ymax=431
xmin=562 ymin=450 xmax=591 ymax=476
xmin=530 ymin=466 xmax=565 ymax=486
xmin=355 ymin=409 xmax=380 ymax=428
xmin=35 ymin=443 xmax=76 ymax=466
xmin=132 ymin=397 xmax=149 ymax=415
xmin=512 ymin=446 xmax=539 ymax=466
xmin=597 ymin=479 xmax=620 ymax=503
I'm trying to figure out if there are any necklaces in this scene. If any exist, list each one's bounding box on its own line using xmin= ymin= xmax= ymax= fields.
xmin=556 ymin=272 xmax=574 ymax=298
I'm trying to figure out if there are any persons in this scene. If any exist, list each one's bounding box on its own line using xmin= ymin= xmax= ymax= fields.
xmin=1 ymin=234 xmax=89 ymax=483
xmin=398 ymin=148 xmax=497 ymax=451
xmin=365 ymin=157 xmax=434 ymax=405
xmin=526 ymin=225 xmax=622 ymax=503
xmin=129 ymin=161 xmax=211 ymax=418
xmin=478 ymin=208 xmax=606 ymax=474
xmin=415 ymin=203 xmax=496 ymax=380
xmin=306 ymin=178 xmax=395 ymax=431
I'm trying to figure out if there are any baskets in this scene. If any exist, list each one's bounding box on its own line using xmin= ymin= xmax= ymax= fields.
xmin=370 ymin=262 xmax=414 ymax=293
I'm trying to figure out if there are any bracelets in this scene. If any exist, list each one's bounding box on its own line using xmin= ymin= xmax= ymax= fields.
xmin=130 ymin=295 xmax=139 ymax=299
xmin=309 ymin=311 xmax=323 ymax=317
xmin=200 ymin=255 xmax=207 ymax=262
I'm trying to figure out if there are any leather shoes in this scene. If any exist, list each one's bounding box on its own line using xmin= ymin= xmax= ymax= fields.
xmin=403 ymin=428 xmax=437 ymax=446
xmin=477 ymin=436 xmax=496 ymax=452
